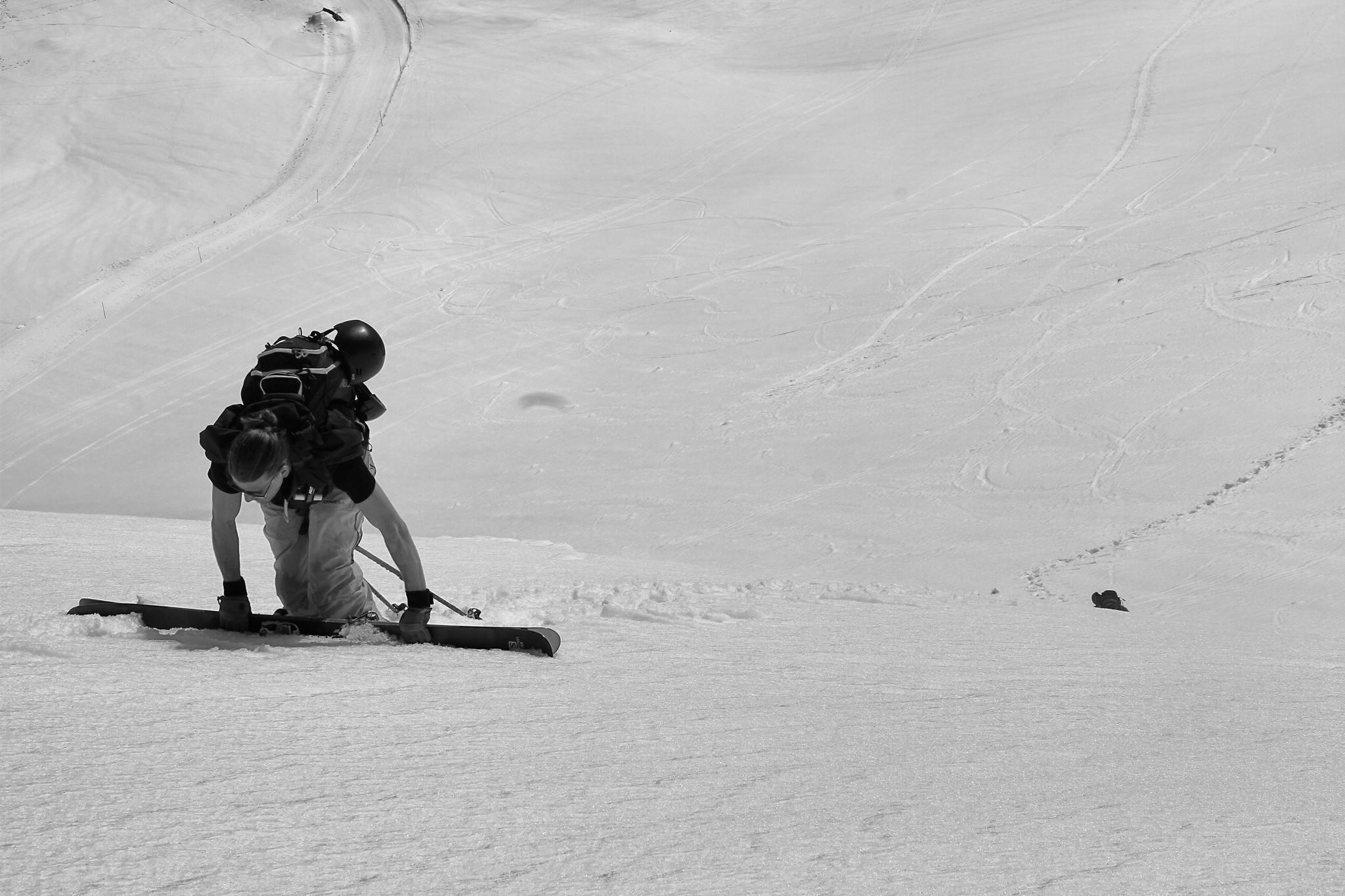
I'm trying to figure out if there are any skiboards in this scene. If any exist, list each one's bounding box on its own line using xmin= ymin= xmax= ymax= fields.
xmin=65 ymin=596 xmax=562 ymax=658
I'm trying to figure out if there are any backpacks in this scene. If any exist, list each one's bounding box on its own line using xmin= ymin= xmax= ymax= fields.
xmin=241 ymin=329 xmax=387 ymax=422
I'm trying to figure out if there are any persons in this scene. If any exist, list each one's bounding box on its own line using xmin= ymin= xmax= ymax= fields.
xmin=1091 ymin=591 xmax=1129 ymax=612
xmin=199 ymin=393 xmax=433 ymax=644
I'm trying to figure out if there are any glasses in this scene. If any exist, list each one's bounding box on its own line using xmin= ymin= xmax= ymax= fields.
xmin=228 ymin=478 xmax=272 ymax=499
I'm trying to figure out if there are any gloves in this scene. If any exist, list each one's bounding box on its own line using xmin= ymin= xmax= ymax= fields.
xmin=218 ymin=596 xmax=255 ymax=635
xmin=400 ymin=607 xmax=431 ymax=644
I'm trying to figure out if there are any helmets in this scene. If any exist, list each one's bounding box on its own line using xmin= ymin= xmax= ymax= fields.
xmin=335 ymin=320 xmax=385 ymax=386
